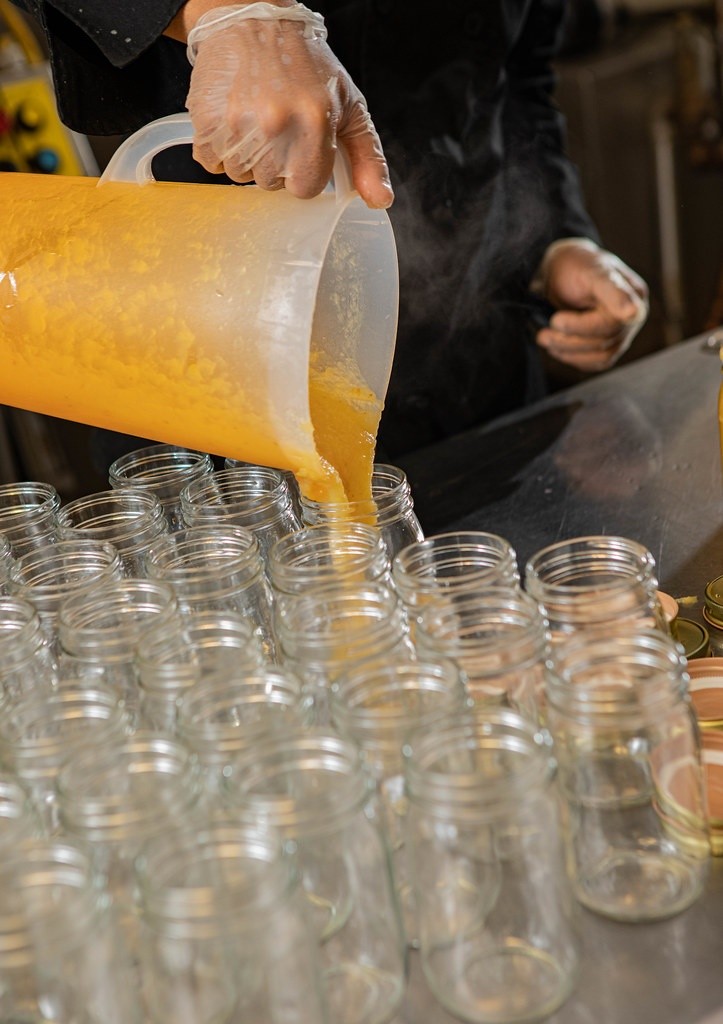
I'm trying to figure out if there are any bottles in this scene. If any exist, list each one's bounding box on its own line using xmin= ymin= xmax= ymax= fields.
xmin=523 ymin=530 xmax=673 ymax=812
xmin=542 ymin=626 xmax=715 ymax=919
xmin=0 ymin=0 xmax=85 ymax=178
xmin=2 ymin=445 xmax=576 ymax=1023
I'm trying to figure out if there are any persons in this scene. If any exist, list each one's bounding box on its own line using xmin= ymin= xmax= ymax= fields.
xmin=9 ymin=0 xmax=650 ymax=467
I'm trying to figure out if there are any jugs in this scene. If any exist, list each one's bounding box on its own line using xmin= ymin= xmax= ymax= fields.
xmin=0 ymin=112 xmax=398 ymax=515
